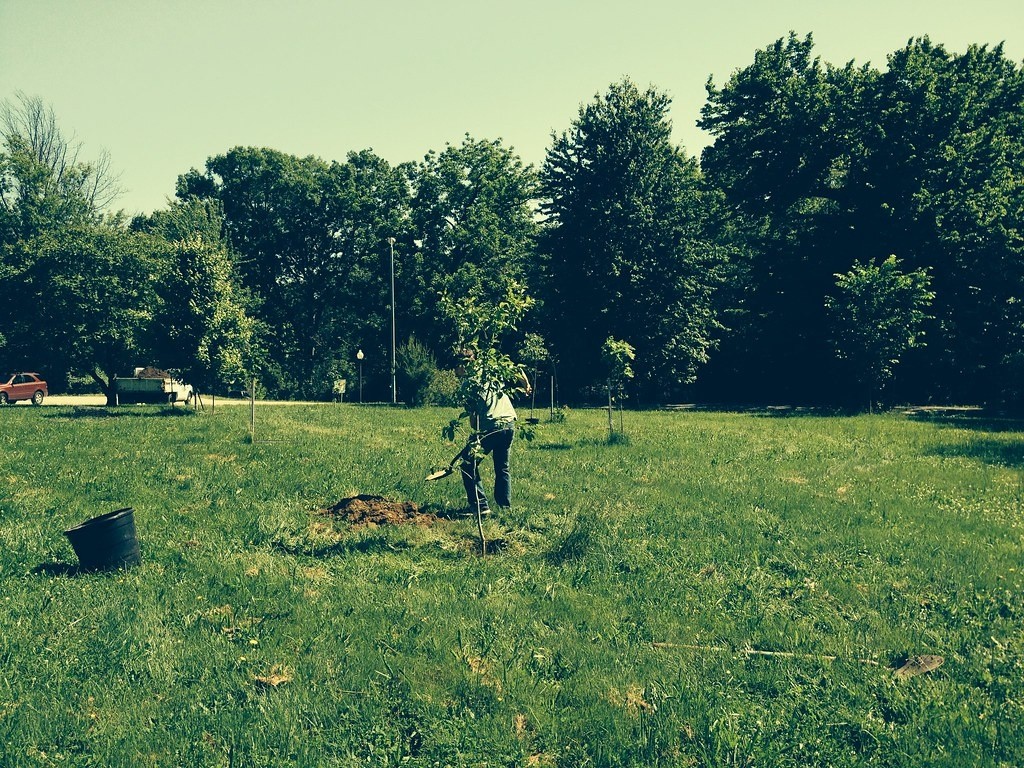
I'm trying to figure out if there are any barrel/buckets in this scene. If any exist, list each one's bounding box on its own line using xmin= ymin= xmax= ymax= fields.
xmin=64 ymin=506 xmax=139 ymax=571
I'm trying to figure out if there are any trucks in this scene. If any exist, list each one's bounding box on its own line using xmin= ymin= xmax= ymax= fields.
xmin=114 ymin=367 xmax=193 ymax=406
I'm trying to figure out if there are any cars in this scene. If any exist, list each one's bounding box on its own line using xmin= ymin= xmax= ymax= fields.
xmin=0 ymin=373 xmax=48 ymax=405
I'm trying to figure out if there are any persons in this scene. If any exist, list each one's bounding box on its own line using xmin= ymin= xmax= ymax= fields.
xmin=457 ymin=351 xmax=518 ymax=516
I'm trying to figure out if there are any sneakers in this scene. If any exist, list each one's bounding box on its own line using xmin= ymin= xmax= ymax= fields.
xmin=458 ymin=506 xmax=490 ymax=515
